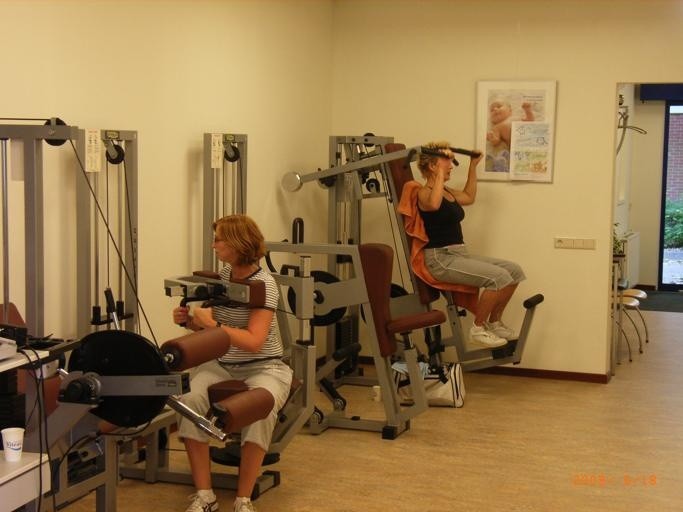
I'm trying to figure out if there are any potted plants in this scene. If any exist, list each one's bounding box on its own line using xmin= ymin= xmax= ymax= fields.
xmin=475 ymin=79 xmax=558 ymax=183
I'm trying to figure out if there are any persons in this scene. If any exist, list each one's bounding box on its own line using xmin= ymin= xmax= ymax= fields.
xmin=417 ymin=141 xmax=527 ymax=348
xmin=173 ymin=215 xmax=294 ymax=512
xmin=487 ymin=100 xmax=534 ymax=147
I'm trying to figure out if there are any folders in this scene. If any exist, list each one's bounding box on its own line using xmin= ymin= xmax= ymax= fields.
xmin=621 ymin=231 xmax=640 ymax=288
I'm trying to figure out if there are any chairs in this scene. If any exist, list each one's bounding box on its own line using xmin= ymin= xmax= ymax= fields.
xmin=468 ymin=329 xmax=506 ymax=347
xmin=234 ymin=496 xmax=254 ymax=511
xmin=485 ymin=319 xmax=519 ymax=340
xmin=186 ymin=493 xmax=219 ymax=512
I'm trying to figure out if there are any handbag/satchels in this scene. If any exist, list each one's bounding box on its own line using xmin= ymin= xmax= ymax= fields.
xmin=216 ymin=319 xmax=221 ymax=329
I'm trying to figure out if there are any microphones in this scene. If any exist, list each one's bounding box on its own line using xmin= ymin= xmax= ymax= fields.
xmin=385 ymin=310 xmax=445 ymax=423
xmin=207 ymin=378 xmax=250 ymax=443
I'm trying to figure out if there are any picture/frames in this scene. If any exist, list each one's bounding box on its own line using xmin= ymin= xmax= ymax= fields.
xmin=0 ymin=349 xmax=51 ymax=512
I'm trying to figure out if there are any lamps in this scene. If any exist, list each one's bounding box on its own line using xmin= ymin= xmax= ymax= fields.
xmin=612 ymin=289 xmax=649 ymax=353
xmin=384 ymin=137 xmax=484 ymax=362
xmin=612 ymin=254 xmax=628 ymax=364
xmin=610 ymin=297 xmax=641 ymax=362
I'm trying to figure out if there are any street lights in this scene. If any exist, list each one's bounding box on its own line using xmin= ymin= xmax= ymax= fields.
xmin=392 ymin=362 xmax=466 ymax=407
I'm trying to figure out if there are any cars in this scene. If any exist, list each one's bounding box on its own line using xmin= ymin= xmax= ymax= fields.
xmin=0 ymin=427 xmax=24 ymax=463
